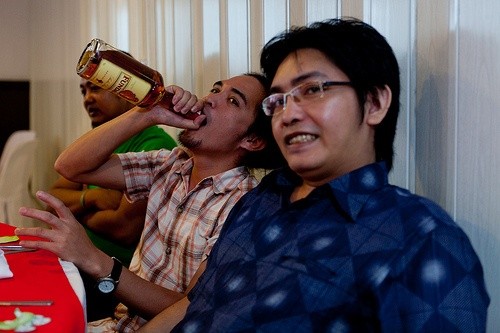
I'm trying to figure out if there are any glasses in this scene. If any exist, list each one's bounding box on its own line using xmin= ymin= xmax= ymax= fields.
xmin=261 ymin=79 xmax=373 ymax=116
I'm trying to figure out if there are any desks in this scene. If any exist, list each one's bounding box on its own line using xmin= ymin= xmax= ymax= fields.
xmin=0 ymin=222 xmax=87 ymax=333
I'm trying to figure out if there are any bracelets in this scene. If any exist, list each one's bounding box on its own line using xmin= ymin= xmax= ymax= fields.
xmin=80 ymin=189 xmax=88 ymax=211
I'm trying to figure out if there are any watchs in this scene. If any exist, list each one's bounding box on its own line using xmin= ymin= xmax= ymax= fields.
xmin=93 ymin=256 xmax=122 ymax=295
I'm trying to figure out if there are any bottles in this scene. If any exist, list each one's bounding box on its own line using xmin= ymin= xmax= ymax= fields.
xmin=75 ymin=37 xmax=203 ymax=123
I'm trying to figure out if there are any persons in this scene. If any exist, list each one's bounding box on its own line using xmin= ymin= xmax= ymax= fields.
xmin=45 ymin=50 xmax=179 ymax=322
xmin=14 ymin=72 xmax=287 ymax=333
xmin=133 ymin=15 xmax=491 ymax=333
